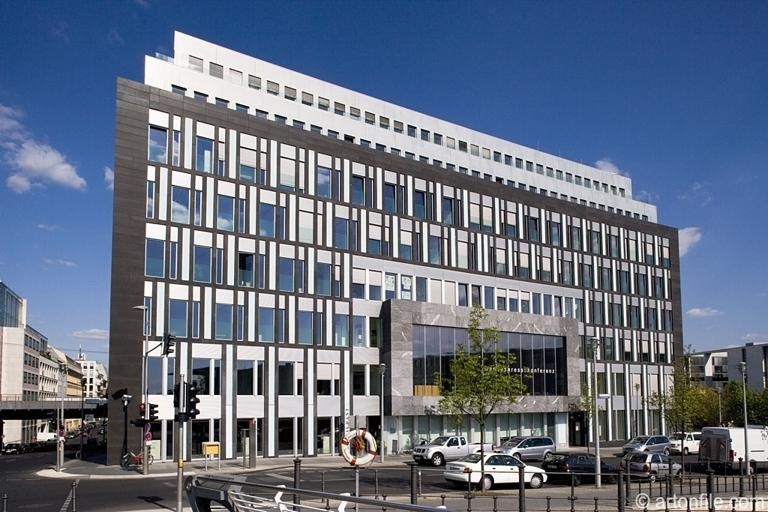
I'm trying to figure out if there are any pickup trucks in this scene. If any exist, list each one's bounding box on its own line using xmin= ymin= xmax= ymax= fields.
xmin=412 ymin=436 xmax=493 ymax=467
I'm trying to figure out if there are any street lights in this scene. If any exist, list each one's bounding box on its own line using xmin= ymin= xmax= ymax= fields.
xmin=380 ymin=362 xmax=385 ymax=463
xmin=735 ymin=361 xmax=751 ymax=476
xmin=587 ymin=338 xmax=600 ymax=488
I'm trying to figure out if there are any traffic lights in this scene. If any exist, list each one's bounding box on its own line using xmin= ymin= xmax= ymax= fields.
xmin=149 ymin=403 xmax=158 ymax=422
xmin=139 ymin=403 xmax=145 ymax=416
xmin=161 ymin=334 xmax=176 ymax=357
xmin=185 ymin=383 xmax=200 ymax=420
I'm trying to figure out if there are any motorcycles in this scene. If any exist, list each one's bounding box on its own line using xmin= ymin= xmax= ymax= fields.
xmin=121 ymin=445 xmax=153 ymax=471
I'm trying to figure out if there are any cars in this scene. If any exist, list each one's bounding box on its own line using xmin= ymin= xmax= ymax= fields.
xmin=444 ymin=452 xmax=548 ymax=489
xmin=542 ymin=453 xmax=618 ymax=487
xmin=622 ymin=435 xmax=685 ymax=482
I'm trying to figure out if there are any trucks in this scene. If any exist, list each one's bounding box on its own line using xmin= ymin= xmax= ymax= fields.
xmin=698 ymin=425 xmax=768 ymax=474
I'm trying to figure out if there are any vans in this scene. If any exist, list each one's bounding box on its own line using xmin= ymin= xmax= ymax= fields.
xmin=494 ymin=436 xmax=556 ymax=460
xmin=669 ymin=432 xmax=702 ymax=455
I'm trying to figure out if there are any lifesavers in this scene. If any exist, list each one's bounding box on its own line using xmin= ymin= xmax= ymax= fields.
xmin=341 ymin=430 xmax=377 ymax=466
